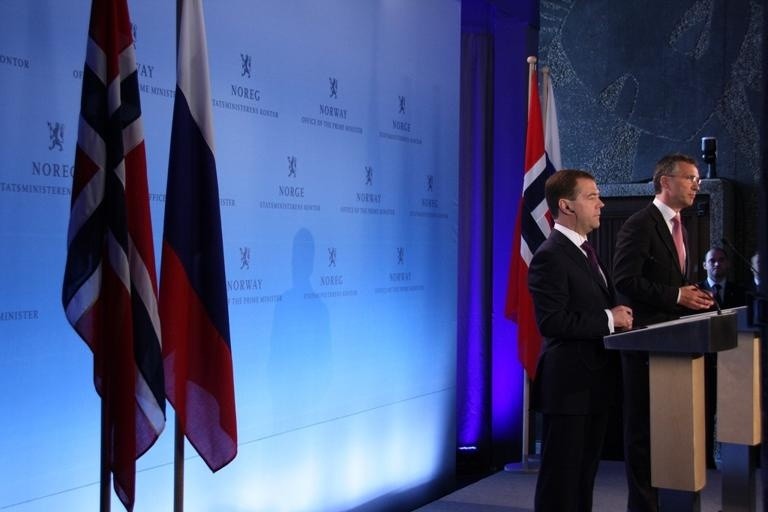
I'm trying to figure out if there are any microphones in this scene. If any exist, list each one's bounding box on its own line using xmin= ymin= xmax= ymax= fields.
xmin=640 ymin=251 xmax=721 ymax=315
xmin=720 ymin=237 xmax=768 ymax=296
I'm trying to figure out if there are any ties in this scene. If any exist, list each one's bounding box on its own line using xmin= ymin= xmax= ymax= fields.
xmin=671 ymin=216 xmax=684 ymax=273
xmin=580 ymin=241 xmax=598 ymax=273
xmin=711 ymin=283 xmax=722 ymax=304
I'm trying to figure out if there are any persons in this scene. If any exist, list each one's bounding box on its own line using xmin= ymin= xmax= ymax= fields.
xmin=527 ymin=169 xmax=634 ymax=512
xmin=689 ymin=247 xmax=746 ymax=311
xmin=750 ymin=252 xmax=768 ymax=329
xmin=610 ymin=153 xmax=715 ymax=512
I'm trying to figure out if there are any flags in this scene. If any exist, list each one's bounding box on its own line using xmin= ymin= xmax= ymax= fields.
xmin=544 ymin=73 xmax=562 ymax=173
xmin=158 ymin=0 xmax=237 ymax=475
xmin=505 ymin=65 xmax=556 ymax=383
xmin=61 ymin=1 xmax=166 ymax=512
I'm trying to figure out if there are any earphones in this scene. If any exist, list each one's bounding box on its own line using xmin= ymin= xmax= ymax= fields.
xmin=566 ymin=205 xmax=576 ymax=214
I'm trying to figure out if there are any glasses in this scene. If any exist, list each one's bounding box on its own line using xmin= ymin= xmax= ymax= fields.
xmin=664 ymin=174 xmax=702 ymax=183
